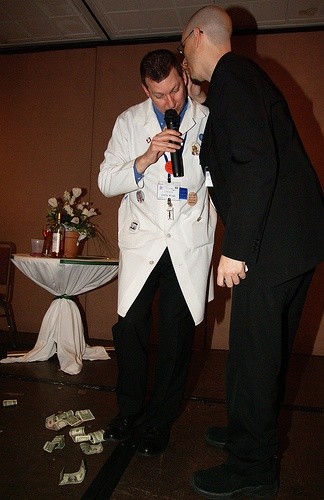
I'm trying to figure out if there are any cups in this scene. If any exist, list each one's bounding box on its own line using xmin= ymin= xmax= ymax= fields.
xmin=30 ymin=238 xmax=45 ymax=256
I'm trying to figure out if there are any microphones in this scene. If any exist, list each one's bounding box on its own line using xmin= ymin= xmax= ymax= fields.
xmin=164 ymin=109 xmax=184 ymax=178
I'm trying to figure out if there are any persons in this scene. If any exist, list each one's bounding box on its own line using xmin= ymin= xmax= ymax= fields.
xmin=177 ymin=7 xmax=324 ymax=496
xmin=97 ymin=49 xmax=218 ymax=457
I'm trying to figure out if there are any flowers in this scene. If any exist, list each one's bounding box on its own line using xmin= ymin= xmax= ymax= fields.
xmin=45 ymin=186 xmax=97 ymax=240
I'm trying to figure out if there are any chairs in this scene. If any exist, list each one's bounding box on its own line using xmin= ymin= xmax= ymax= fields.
xmin=0 ymin=242 xmax=17 ymax=348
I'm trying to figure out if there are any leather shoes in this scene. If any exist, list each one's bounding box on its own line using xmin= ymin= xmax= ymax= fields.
xmin=103 ymin=413 xmax=142 ymax=441
xmin=139 ymin=419 xmax=172 ymax=456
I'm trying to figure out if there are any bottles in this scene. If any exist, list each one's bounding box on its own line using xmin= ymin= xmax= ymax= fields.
xmin=51 ymin=210 xmax=64 ymax=258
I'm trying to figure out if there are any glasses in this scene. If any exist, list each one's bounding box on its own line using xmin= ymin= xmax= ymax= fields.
xmin=178 ymin=30 xmax=202 ymax=56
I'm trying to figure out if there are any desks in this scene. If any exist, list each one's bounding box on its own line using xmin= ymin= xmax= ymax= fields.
xmin=0 ymin=252 xmax=118 ymax=376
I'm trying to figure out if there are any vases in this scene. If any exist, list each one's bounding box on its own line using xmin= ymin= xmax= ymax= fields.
xmin=63 ymin=231 xmax=80 ymax=258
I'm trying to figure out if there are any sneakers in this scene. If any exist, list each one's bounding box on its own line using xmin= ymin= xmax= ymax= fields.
xmin=187 ymin=461 xmax=280 ymax=500
xmin=205 ymin=425 xmax=229 ymax=448
